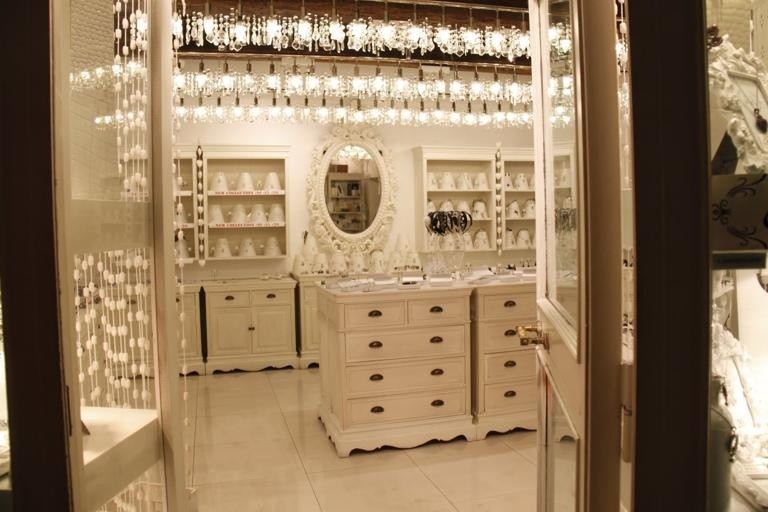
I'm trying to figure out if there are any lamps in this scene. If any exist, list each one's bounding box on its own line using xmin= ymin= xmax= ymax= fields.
xmin=51 ymin=3 xmax=584 ymax=131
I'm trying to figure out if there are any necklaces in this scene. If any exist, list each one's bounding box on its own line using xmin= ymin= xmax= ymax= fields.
xmin=731 ymin=74 xmax=768 ymax=137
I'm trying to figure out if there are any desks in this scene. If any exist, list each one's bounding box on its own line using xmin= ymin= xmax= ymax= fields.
xmin=22 ymin=398 xmax=169 ymax=502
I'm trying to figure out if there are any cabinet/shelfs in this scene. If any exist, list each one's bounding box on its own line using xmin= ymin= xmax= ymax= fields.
xmin=93 ymin=137 xmax=633 ymax=450
